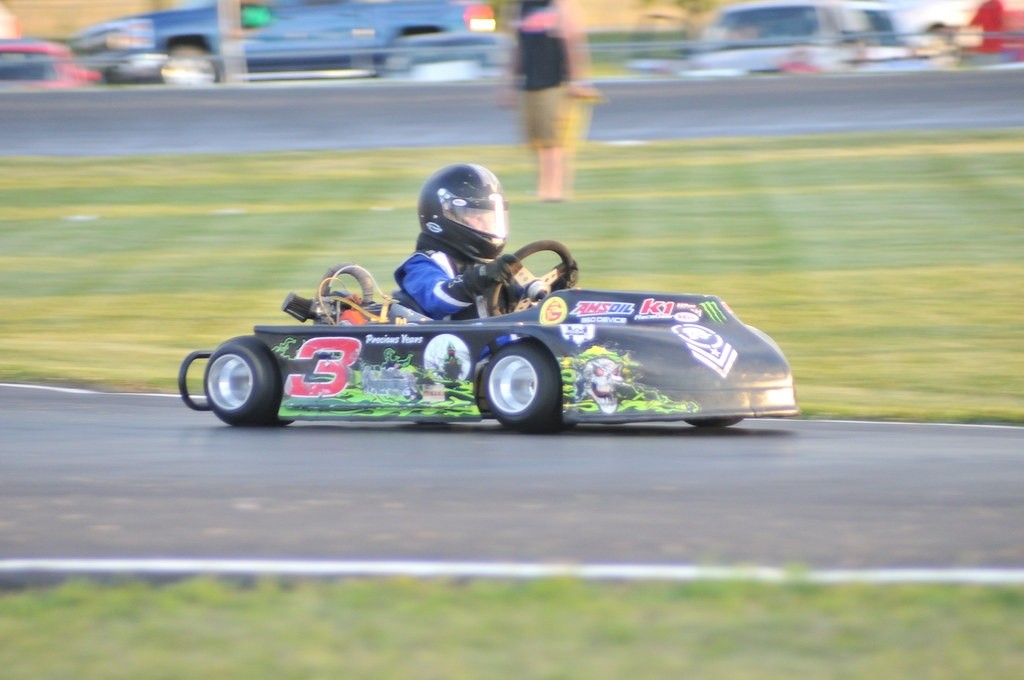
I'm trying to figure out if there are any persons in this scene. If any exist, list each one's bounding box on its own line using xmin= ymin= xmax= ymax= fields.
xmin=394 ymin=162 xmax=545 ymax=321
xmin=501 ymin=0 xmax=607 ymax=199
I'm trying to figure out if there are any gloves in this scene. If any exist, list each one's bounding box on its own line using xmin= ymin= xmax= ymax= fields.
xmin=463 ymin=254 xmax=516 ymax=296
xmin=551 ymin=259 xmax=579 ymax=292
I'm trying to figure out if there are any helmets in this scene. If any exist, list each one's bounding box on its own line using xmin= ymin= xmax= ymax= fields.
xmin=416 ymin=163 xmax=506 ymax=263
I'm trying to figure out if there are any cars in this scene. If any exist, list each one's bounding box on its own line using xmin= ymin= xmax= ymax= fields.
xmin=69 ymin=1 xmax=498 ymax=85
xmin=680 ymin=1 xmax=1024 ymax=69
xmin=1 ymin=41 xmax=102 ymax=90
